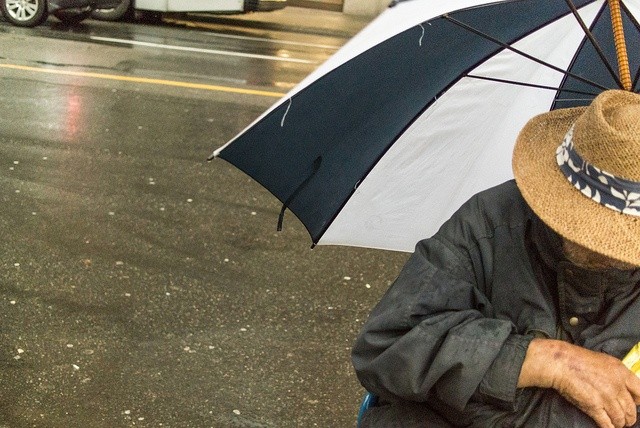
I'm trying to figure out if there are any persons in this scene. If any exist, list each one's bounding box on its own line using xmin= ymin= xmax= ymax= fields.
xmin=351 ymin=87 xmax=639 ymax=428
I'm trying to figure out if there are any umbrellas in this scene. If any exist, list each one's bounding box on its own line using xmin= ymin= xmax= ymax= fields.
xmin=205 ymin=1 xmax=639 ymax=255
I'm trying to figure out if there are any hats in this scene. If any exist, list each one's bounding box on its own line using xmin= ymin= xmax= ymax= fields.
xmin=512 ymin=89 xmax=640 ymax=266
